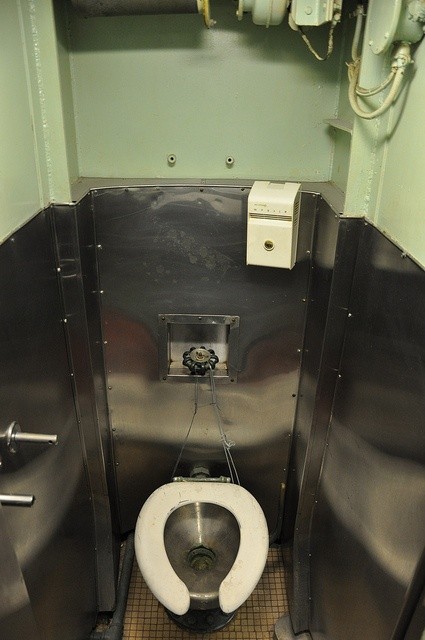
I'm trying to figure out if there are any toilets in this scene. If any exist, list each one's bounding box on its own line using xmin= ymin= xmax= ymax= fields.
xmin=134 ymin=481 xmax=269 ymax=633
